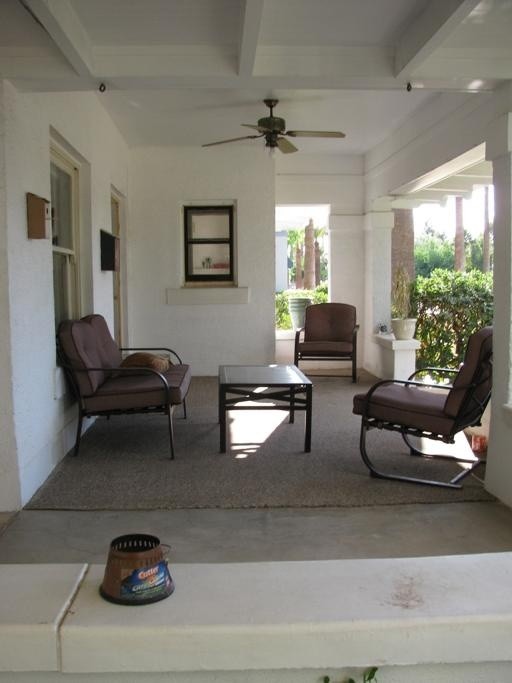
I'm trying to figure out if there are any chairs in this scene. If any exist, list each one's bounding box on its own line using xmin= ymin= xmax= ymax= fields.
xmin=353 ymin=325 xmax=493 ymax=489
xmin=294 ymin=302 xmax=360 ymax=382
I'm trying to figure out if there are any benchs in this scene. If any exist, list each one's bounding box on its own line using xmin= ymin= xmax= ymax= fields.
xmin=58 ymin=313 xmax=191 ymax=459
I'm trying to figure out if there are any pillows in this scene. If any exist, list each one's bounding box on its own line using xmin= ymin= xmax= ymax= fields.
xmin=111 ymin=352 xmax=170 ymax=379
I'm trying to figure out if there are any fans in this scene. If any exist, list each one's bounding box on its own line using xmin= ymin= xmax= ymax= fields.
xmin=201 ymin=99 xmax=345 ymax=153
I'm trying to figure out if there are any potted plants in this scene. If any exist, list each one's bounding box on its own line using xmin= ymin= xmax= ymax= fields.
xmin=390 ymin=258 xmax=418 ymax=339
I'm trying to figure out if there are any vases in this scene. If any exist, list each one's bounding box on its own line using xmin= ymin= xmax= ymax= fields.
xmin=288 ymin=298 xmax=312 ymax=328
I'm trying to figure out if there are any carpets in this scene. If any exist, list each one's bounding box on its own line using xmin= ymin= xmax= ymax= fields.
xmin=21 ymin=367 xmax=500 ymax=510
